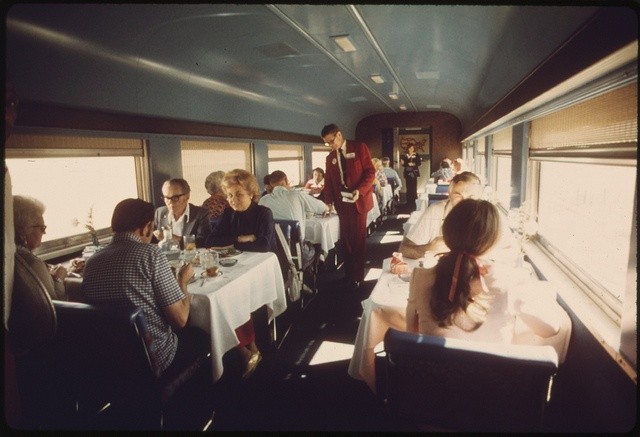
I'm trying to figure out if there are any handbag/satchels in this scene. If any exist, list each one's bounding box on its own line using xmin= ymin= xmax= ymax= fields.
xmin=275 ymin=223 xmax=303 ymax=301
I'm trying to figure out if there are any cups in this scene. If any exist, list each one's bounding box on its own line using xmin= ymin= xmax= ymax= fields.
xmin=183 ymin=234 xmax=196 ymax=257
xmin=205 ymin=252 xmax=219 ymax=270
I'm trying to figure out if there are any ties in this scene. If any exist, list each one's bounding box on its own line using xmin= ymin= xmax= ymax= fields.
xmin=338 ymin=147 xmax=348 ymax=187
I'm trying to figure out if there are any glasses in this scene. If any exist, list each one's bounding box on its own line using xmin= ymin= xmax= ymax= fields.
xmin=324 ymin=134 xmax=337 ymax=147
xmin=30 ymin=224 xmax=47 ymax=231
xmin=161 ymin=193 xmax=185 ymax=202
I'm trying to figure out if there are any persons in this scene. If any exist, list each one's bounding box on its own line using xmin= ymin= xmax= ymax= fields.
xmin=430 ymin=157 xmax=464 ymax=183
xmin=150 ymin=177 xmax=210 ymax=250
xmin=201 ymin=169 xmax=228 ymax=218
xmin=320 ymin=123 xmax=377 ymax=292
xmin=304 ymin=167 xmax=325 ymax=193
xmin=398 ymin=171 xmax=483 ymax=260
xmin=257 ymin=170 xmax=331 ymax=295
xmin=370 ymin=155 xmax=402 ymax=210
xmin=13 ymin=194 xmax=69 ymax=301
xmin=259 ymin=173 xmax=274 ymax=197
xmin=399 ymin=142 xmax=422 ymax=207
xmin=77 ymin=197 xmax=197 ymax=385
xmin=203 ymin=168 xmax=290 ymax=379
xmin=404 ymin=198 xmax=562 ymax=365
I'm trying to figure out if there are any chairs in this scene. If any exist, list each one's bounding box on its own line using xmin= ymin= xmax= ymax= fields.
xmin=371 ymin=184 xmax=384 ymax=230
xmin=436 ymin=185 xmax=450 ymax=193
xmin=53 ymin=300 xmax=213 ymax=437
xmin=428 ymin=194 xmax=448 ymax=207
xmin=386 ymin=177 xmax=395 ymax=216
xmin=272 ymin=220 xmax=319 ymax=314
xmin=373 ymin=327 xmax=558 ymax=436
xmin=208 ymin=218 xmax=294 ymax=342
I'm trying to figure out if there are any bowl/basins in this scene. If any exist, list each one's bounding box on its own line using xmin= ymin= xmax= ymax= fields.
xmin=163 ymin=250 xmax=180 ymax=260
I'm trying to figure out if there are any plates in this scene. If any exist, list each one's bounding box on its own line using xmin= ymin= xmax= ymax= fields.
xmin=213 ymin=248 xmax=243 ymax=259
xmin=200 ymin=271 xmax=223 ymax=279
